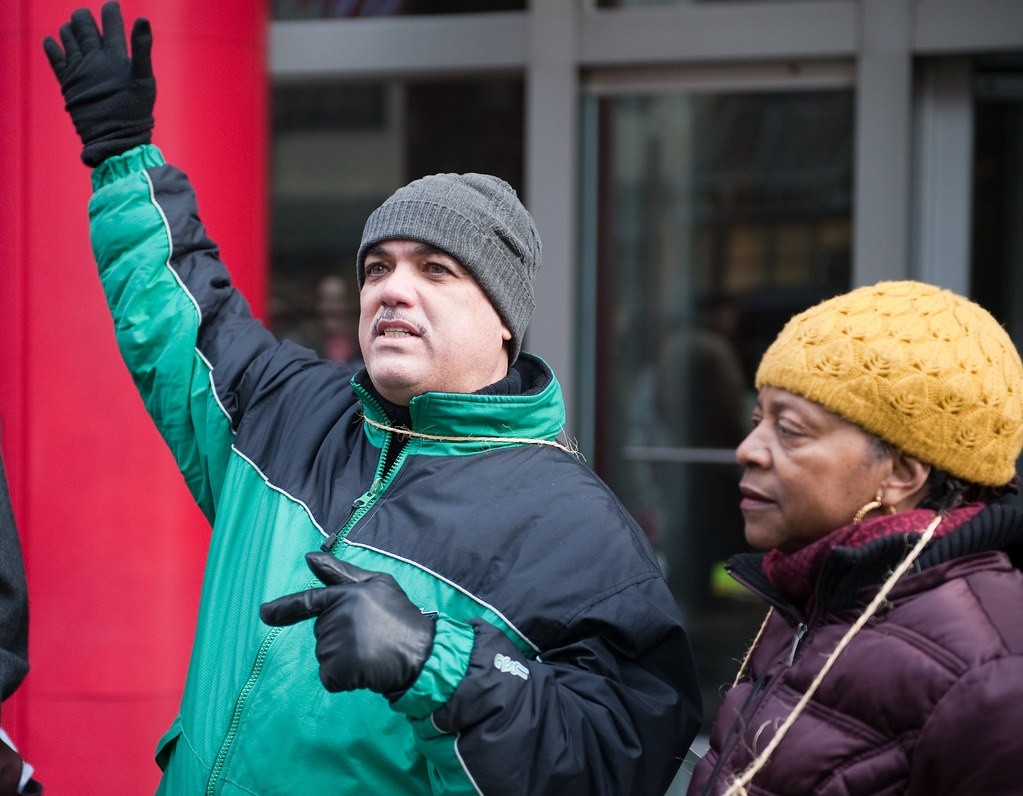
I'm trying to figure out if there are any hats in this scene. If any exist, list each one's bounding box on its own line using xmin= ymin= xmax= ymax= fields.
xmin=355 ymin=172 xmax=543 ymax=370
xmin=754 ymin=279 xmax=1023 ymax=489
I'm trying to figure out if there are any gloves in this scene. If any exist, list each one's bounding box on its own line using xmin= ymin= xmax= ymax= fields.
xmin=259 ymin=551 xmax=435 ymax=697
xmin=43 ymin=1 xmax=157 ymax=168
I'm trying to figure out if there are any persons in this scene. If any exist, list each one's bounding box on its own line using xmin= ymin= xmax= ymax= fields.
xmin=643 ymin=283 xmax=760 ymax=607
xmin=44 ymin=3 xmax=700 ymax=796
xmin=0 ymin=455 xmax=41 ymax=796
xmin=284 ymin=277 xmax=358 ymax=363
xmin=684 ymin=279 xmax=1023 ymax=796
